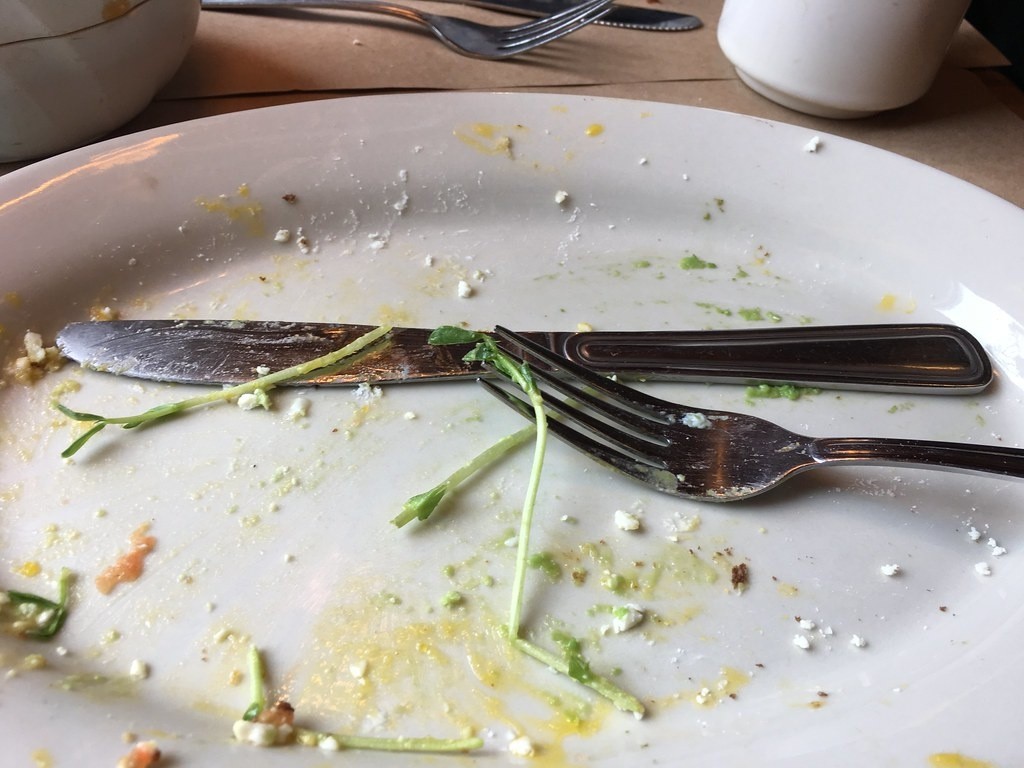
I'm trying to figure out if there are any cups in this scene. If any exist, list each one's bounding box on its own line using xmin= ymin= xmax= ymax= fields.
xmin=716 ymin=1 xmax=970 ymax=118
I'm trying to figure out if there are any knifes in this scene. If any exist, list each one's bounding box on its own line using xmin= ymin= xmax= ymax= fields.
xmin=431 ymin=0 xmax=706 ymax=32
xmin=55 ymin=318 xmax=994 ymax=396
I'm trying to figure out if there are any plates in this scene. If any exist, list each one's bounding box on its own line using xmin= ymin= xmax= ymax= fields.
xmin=2 ymin=92 xmax=1022 ymax=767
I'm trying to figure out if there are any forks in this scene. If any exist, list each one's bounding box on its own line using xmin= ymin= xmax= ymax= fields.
xmin=476 ymin=323 xmax=1024 ymax=505
xmin=201 ymin=0 xmax=622 ymax=61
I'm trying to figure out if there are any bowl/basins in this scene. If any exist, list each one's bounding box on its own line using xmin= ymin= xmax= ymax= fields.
xmin=1 ymin=1 xmax=202 ymax=165
